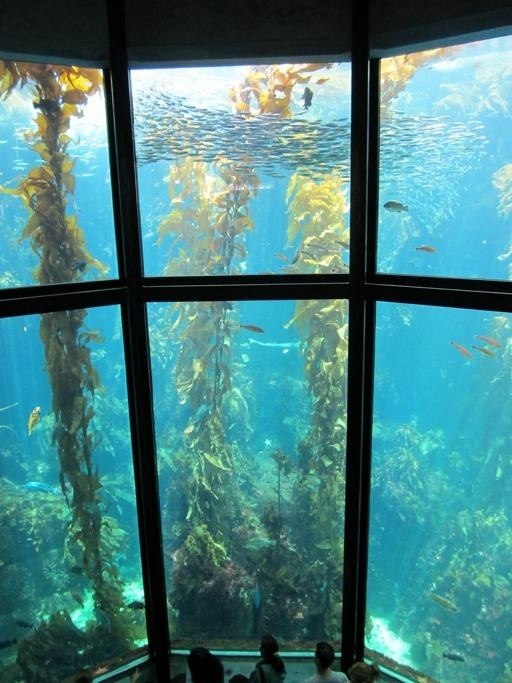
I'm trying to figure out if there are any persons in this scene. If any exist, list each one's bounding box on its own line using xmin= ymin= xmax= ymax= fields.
xmin=188 ymin=635 xmax=379 ymax=682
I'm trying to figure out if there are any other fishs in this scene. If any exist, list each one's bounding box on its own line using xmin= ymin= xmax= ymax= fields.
xmin=428 ymin=592 xmax=459 ymax=615
xmin=71 ymin=565 xmax=83 ymax=573
xmin=416 ymin=244 xmax=437 ymax=254
xmin=241 ymin=324 xmax=265 ymax=335
xmin=135 ymin=82 xmax=495 ymax=195
xmin=242 ymin=538 xmax=277 ymax=551
xmin=383 ymin=200 xmax=409 ymax=214
xmin=15 ymin=619 xmax=33 ymax=629
xmin=18 ymin=480 xmax=64 ymax=496
xmin=70 ymin=591 xmax=84 ymax=609
xmin=0 ymin=637 xmax=18 ymax=648
xmin=442 ymin=652 xmax=465 ymax=664
xmin=27 ymin=406 xmax=41 ymax=437
xmin=450 ymin=334 xmax=503 ymax=359
